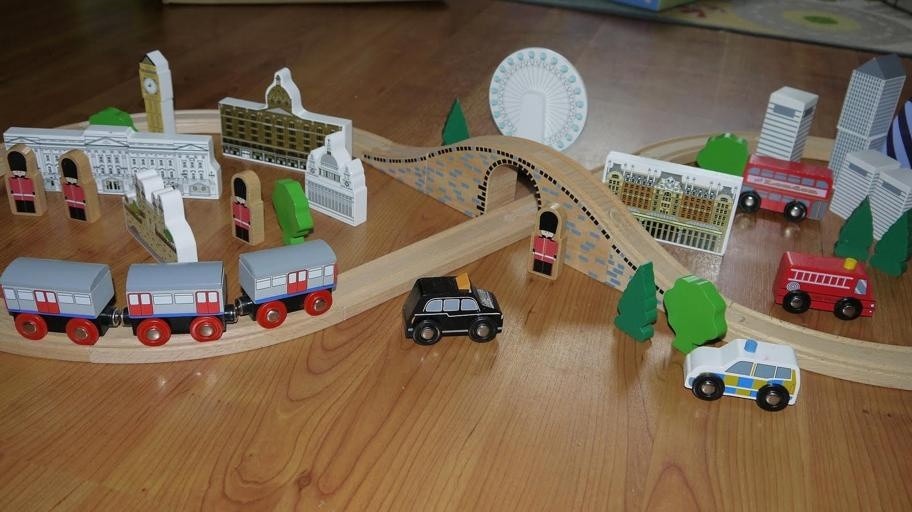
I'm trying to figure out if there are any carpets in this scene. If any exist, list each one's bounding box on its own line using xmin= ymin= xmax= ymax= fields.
xmin=529 ymin=1 xmax=911 ymax=57
